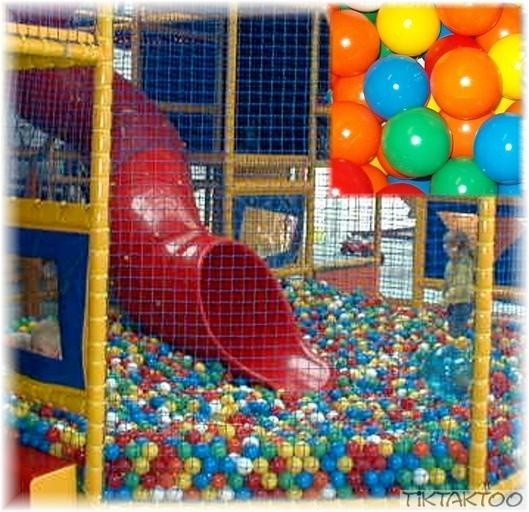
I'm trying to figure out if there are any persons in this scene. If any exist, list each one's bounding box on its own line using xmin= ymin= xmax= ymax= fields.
xmin=443 ymin=230 xmax=474 ymax=338
xmin=3 ymin=275 xmax=525 ymax=506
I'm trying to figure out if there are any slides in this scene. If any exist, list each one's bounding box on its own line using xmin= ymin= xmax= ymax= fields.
xmin=8 ymin=6 xmax=338 ymax=393
